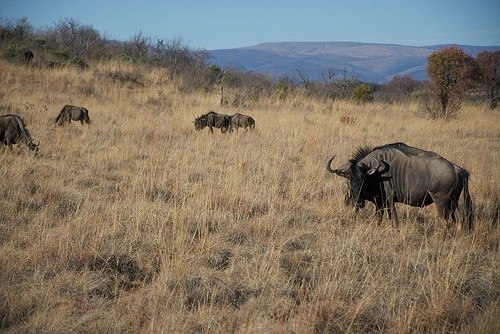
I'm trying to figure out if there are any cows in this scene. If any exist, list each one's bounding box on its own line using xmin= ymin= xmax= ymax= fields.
xmin=0 ymin=114 xmax=40 ymax=156
xmin=193 ymin=111 xmax=229 ymax=134
xmin=229 ymin=114 xmax=255 ymax=133
xmin=55 ymin=105 xmax=90 ymax=126
xmin=326 ymin=142 xmax=472 ymax=230
